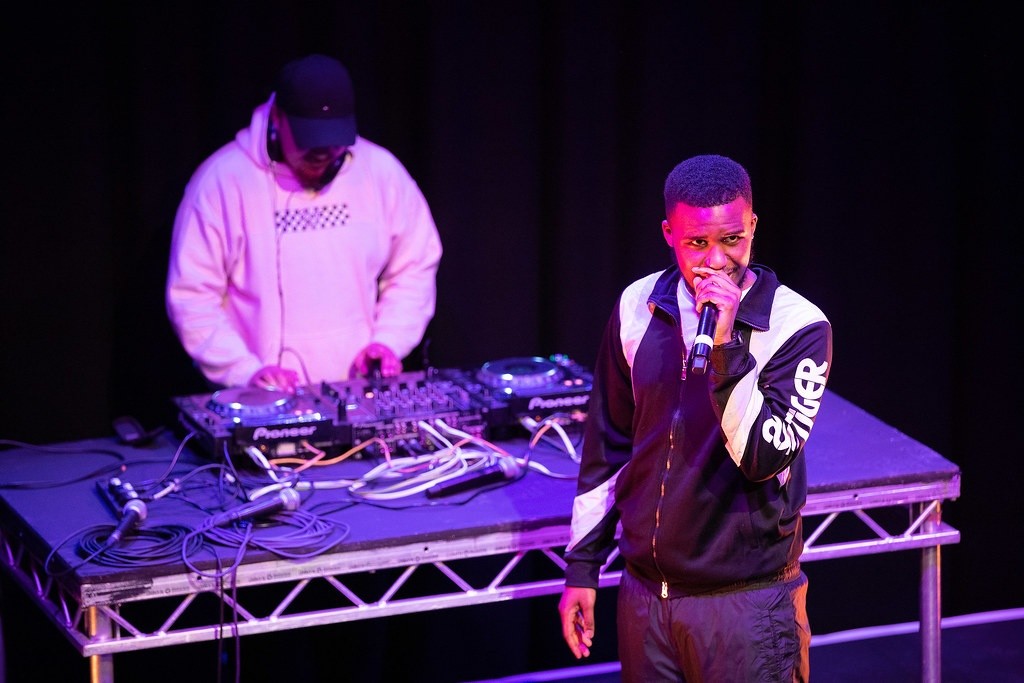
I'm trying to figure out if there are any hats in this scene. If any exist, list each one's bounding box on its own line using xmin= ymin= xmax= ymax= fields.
xmin=278 ymin=55 xmax=357 ymax=149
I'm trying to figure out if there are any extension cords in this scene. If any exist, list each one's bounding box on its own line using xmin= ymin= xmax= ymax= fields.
xmin=96 ymin=479 xmax=127 ymax=519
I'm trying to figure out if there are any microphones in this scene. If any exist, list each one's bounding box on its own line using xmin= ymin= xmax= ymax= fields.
xmin=692 ymin=301 xmax=718 ymax=375
xmin=426 ymin=458 xmax=518 ymax=499
xmin=104 ymin=499 xmax=147 ymax=546
xmin=215 ymin=488 xmax=301 ymax=528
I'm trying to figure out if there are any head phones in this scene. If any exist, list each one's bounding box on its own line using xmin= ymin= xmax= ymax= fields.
xmin=267 ymin=118 xmax=348 ymax=191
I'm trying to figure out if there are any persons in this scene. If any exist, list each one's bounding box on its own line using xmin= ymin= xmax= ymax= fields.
xmin=165 ymin=56 xmax=444 ymax=393
xmin=559 ymin=154 xmax=832 ymax=683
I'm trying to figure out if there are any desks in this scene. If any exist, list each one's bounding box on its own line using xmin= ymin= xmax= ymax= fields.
xmin=0 ymin=387 xmax=961 ymax=683
